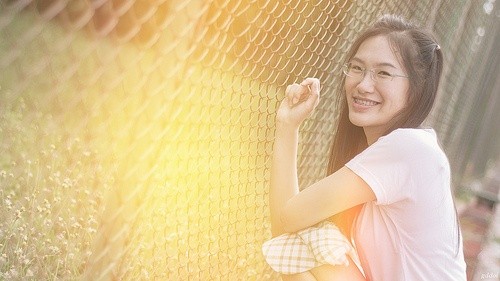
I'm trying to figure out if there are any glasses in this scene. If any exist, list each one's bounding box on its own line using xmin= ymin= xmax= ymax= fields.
xmin=342 ymin=60 xmax=409 ymax=83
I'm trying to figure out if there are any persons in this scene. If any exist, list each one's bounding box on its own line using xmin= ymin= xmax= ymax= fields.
xmin=269 ymin=12 xmax=470 ymax=281
